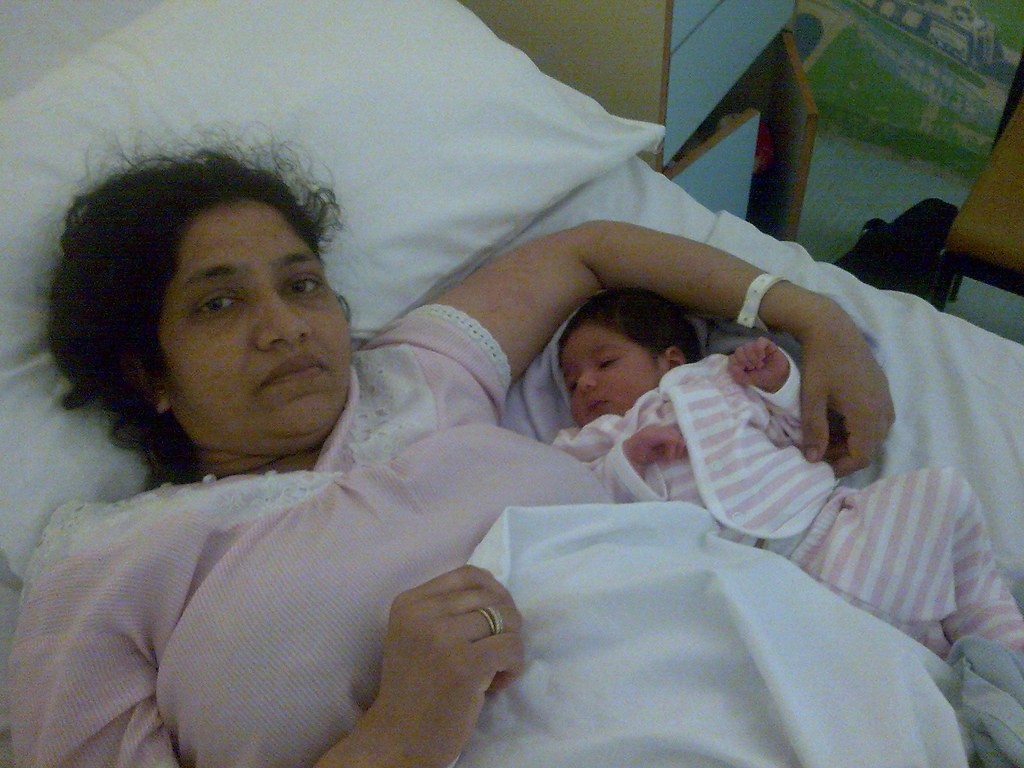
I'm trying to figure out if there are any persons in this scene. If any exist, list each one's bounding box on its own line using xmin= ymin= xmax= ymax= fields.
xmin=1 ymin=154 xmax=1022 ymax=768
xmin=547 ymin=287 xmax=1024 ymax=665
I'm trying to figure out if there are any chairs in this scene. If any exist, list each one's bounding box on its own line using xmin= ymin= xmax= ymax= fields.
xmin=928 ymin=46 xmax=1024 ymax=318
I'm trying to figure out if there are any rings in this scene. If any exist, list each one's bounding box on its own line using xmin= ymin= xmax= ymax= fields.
xmin=477 ymin=607 xmax=503 ymax=634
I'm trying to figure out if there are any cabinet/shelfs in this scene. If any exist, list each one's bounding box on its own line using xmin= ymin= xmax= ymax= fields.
xmin=463 ymin=0 xmax=819 ymax=247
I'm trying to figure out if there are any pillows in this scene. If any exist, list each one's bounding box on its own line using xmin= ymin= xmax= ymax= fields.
xmin=0 ymin=1 xmax=669 ymax=587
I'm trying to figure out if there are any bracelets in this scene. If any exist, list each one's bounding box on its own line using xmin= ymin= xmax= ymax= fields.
xmin=738 ymin=274 xmax=789 ymax=333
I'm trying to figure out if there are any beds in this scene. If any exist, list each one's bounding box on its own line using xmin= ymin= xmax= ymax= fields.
xmin=1 ymin=0 xmax=1024 ymax=768
